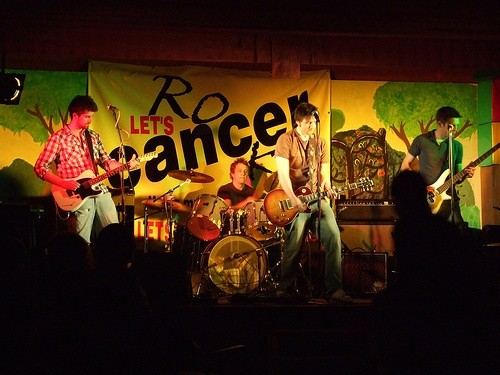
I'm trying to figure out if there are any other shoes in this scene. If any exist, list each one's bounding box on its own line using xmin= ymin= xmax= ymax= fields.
xmin=277 ymin=285 xmax=298 ymax=303
xmin=326 ymin=288 xmax=353 ymax=304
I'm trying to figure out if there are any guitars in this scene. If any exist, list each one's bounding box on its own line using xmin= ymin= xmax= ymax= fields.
xmin=427 ymin=142 xmax=500 ymax=214
xmin=264 ymin=176 xmax=374 ymax=227
xmin=141 ymin=200 xmax=192 ymax=214
xmin=51 ymin=150 xmax=159 ymax=212
xmin=169 ymin=171 xmax=215 ymax=183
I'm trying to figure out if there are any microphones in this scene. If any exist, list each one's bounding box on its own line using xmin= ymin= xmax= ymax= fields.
xmin=312 ymin=111 xmax=320 ymax=122
xmin=107 ymin=105 xmax=120 ymax=112
xmin=234 ymin=254 xmax=240 ymax=259
xmin=448 ymin=124 xmax=455 ymax=131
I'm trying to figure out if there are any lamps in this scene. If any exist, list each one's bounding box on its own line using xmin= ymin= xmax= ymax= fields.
xmin=0 ymin=48 xmax=26 ymax=105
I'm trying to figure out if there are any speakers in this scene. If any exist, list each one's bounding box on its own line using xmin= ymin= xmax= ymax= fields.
xmin=341 ymin=252 xmax=388 ymax=294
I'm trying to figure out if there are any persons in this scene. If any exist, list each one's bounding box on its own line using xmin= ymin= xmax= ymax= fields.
xmin=400 ymin=107 xmax=474 ymax=226
xmin=217 ymin=160 xmax=260 ymax=234
xmin=33 ymin=96 xmax=143 ymax=246
xmin=0 ymin=170 xmax=500 ymax=375
xmin=275 ymin=102 xmax=352 ymax=301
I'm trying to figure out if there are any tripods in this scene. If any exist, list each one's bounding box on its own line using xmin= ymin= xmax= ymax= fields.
xmin=240 ymin=239 xmax=284 ymax=300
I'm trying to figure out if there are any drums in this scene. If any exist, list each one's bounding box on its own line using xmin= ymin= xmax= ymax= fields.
xmin=198 ymin=234 xmax=268 ymax=296
xmin=244 ymin=198 xmax=280 ymax=241
xmin=186 ymin=193 xmax=228 ymax=241
xmin=220 ymin=209 xmax=245 ymax=236
